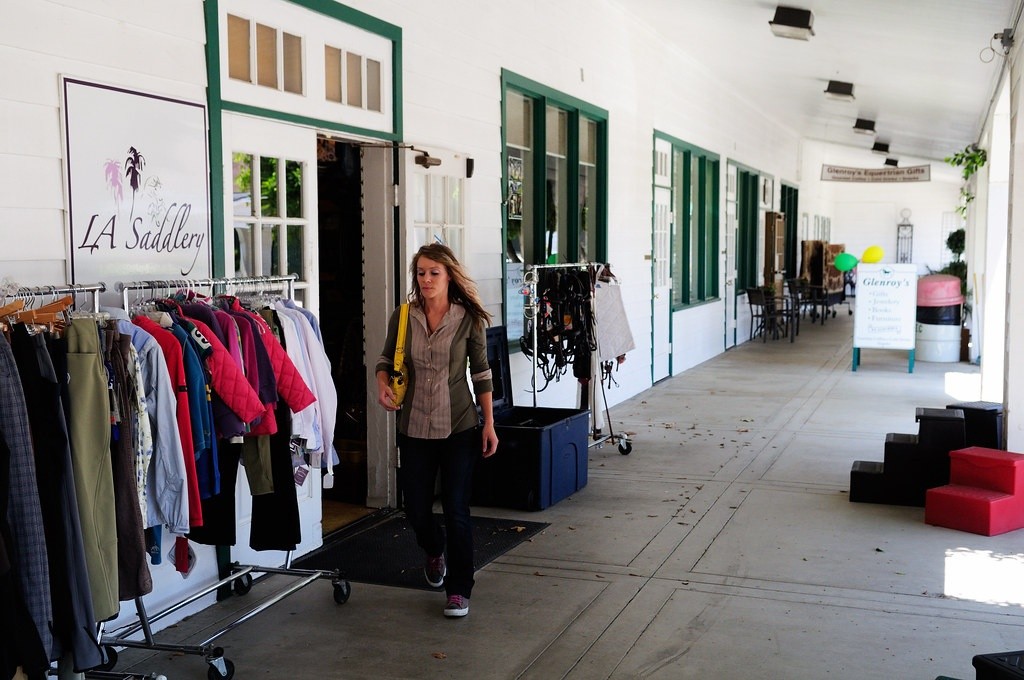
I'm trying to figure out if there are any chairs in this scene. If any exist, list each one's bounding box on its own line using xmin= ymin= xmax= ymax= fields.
xmin=746 ymin=278 xmax=828 ymax=344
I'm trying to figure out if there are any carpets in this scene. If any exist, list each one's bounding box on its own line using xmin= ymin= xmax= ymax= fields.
xmin=291 ymin=512 xmax=552 ymax=592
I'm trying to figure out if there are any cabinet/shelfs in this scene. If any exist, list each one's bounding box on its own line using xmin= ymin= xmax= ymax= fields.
xmin=766 ymin=211 xmax=787 ymax=274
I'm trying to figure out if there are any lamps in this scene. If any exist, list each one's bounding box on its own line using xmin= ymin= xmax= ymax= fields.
xmin=884 ymin=158 xmax=898 ymax=168
xmin=852 ymin=117 xmax=876 ymax=135
xmin=871 ymin=143 xmax=890 ymax=154
xmin=823 ymin=80 xmax=856 ymax=103
xmin=768 ymin=5 xmax=815 ymax=42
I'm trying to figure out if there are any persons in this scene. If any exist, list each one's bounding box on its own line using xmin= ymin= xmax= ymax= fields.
xmin=376 ymin=243 xmax=499 ymax=617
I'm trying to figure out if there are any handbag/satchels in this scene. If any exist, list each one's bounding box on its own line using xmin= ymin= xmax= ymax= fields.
xmin=391 ymin=304 xmax=410 ymax=406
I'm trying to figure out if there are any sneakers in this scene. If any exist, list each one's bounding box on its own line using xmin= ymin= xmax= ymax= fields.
xmin=444 ymin=594 xmax=468 ymax=616
xmin=424 ymin=551 xmax=446 ymax=587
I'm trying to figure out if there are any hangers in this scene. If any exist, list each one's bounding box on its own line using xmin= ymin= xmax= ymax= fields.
xmin=0 ymin=271 xmax=288 ymax=336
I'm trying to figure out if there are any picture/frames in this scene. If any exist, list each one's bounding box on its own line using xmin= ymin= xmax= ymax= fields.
xmin=58 ymin=73 xmax=213 ymax=313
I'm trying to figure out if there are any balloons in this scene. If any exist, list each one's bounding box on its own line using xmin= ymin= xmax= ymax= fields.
xmin=833 ymin=245 xmax=885 ymax=271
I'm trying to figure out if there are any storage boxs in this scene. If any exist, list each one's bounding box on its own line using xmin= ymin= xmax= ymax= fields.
xmin=465 ymin=406 xmax=593 ymax=512
